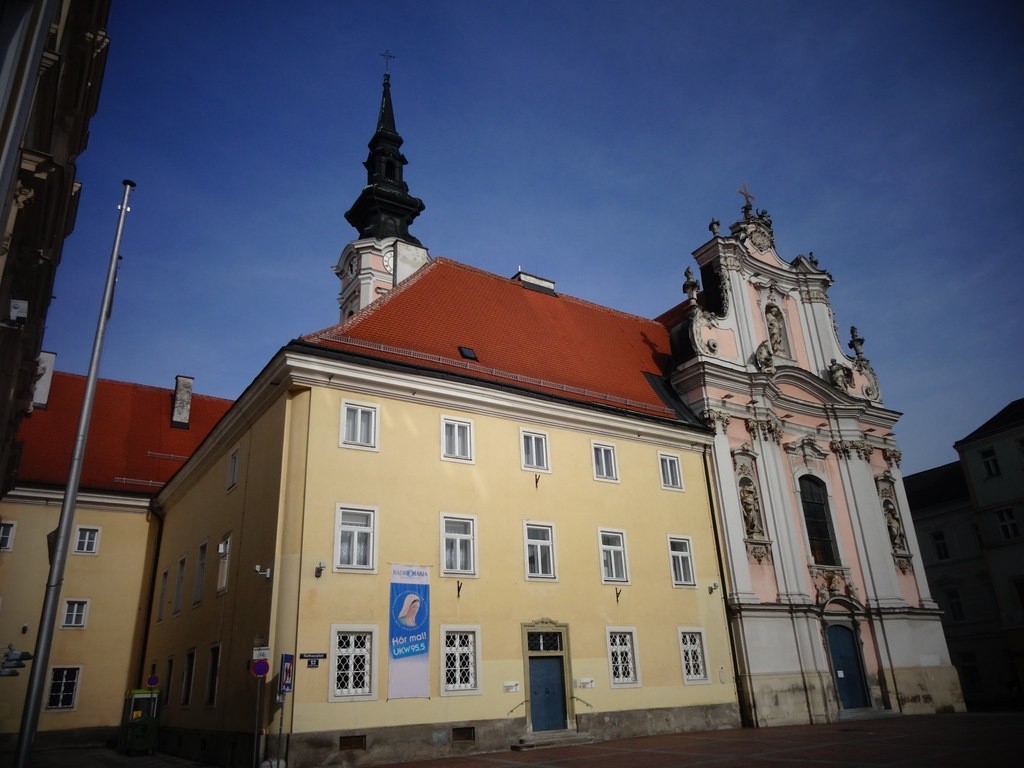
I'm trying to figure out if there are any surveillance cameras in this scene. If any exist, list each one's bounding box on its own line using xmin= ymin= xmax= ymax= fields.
xmin=713 ymin=583 xmax=718 ymax=590
xmin=319 ymin=562 xmax=326 ymax=570
xmin=254 ymin=565 xmax=260 ymax=573
xmin=22 ymin=625 xmax=28 ymax=630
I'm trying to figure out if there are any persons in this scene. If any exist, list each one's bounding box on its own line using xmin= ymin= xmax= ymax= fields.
xmin=766 ymin=307 xmax=784 ymax=355
xmin=756 ymin=339 xmax=775 ymax=369
xmin=884 ymin=504 xmax=907 ymax=550
xmin=738 ymin=481 xmax=764 ymax=535
xmin=829 ymin=359 xmax=850 ymax=389
xmin=397 ymin=594 xmax=420 ymax=627
xmin=755 ymin=206 xmax=771 ymax=225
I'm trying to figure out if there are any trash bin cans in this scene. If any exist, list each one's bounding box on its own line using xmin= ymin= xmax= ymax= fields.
xmin=126 ymin=711 xmax=162 ymax=755
